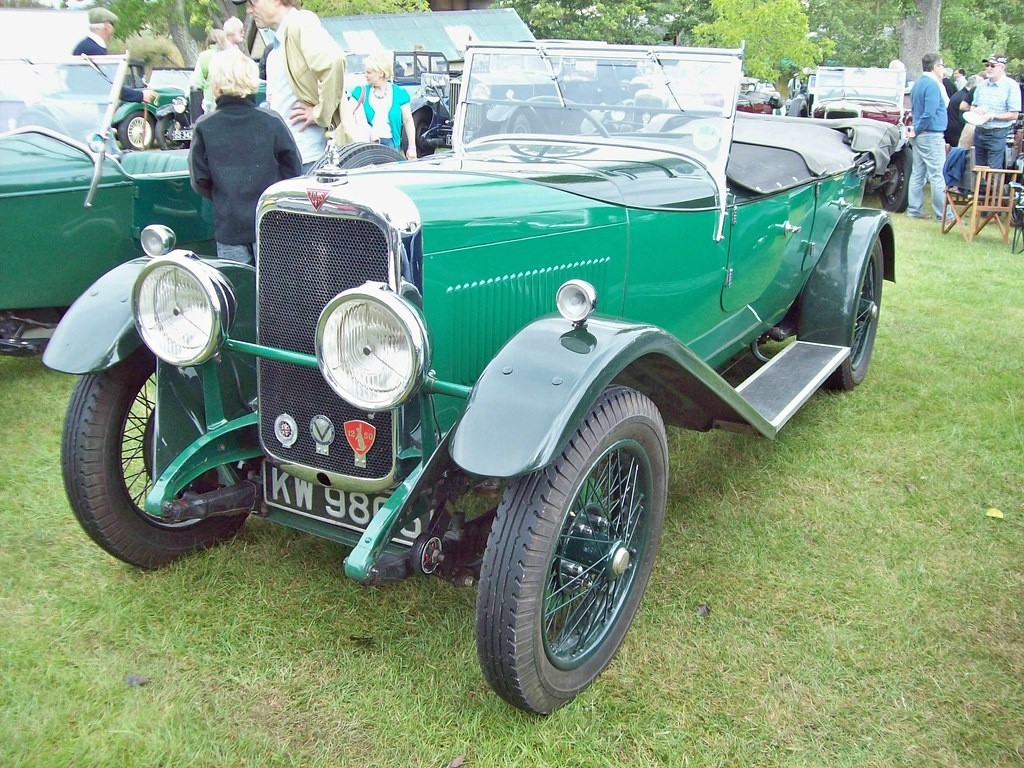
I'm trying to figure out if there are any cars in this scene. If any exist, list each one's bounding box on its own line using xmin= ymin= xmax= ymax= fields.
xmin=38 ymin=42 xmax=899 ymax=720
xmin=0 ymin=6 xmax=912 ymax=364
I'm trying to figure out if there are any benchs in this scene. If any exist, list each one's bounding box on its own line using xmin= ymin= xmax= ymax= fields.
xmin=664 ymin=119 xmax=827 ymax=192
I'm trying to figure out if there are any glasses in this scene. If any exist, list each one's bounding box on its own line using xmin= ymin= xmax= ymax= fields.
xmin=985 ymin=64 xmax=1002 ymax=69
xmin=939 ymin=63 xmax=945 ymax=67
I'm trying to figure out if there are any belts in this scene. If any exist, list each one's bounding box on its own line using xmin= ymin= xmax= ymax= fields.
xmin=976 ymin=128 xmax=1002 ymax=133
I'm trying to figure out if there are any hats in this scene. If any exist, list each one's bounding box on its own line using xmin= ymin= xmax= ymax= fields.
xmin=982 ymin=53 xmax=1009 ymax=65
xmin=89 ymin=7 xmax=120 ymax=24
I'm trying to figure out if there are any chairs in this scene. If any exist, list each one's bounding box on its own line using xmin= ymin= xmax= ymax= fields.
xmin=941 ymin=143 xmax=1022 ymax=245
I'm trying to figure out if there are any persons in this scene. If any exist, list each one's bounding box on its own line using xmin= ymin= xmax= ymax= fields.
xmin=66 ymin=8 xmax=158 ymax=102
xmin=890 ymin=52 xmax=1024 ymax=224
xmin=196 ymin=0 xmax=435 ymax=264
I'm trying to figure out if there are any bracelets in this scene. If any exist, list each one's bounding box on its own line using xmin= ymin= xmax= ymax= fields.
xmin=989 ymin=116 xmax=993 ymax=122
